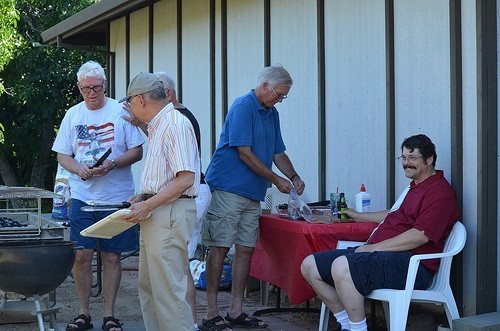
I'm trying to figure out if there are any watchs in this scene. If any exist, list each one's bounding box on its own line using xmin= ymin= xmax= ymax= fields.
xmin=110 ymin=159 xmax=118 ymax=169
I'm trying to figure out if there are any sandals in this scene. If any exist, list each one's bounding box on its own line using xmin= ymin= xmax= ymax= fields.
xmin=102 ymin=316 xmax=124 ymax=331
xmin=202 ymin=315 xmax=232 ymax=331
xmin=66 ymin=314 xmax=93 ymax=331
xmin=225 ymin=312 xmax=268 ymax=329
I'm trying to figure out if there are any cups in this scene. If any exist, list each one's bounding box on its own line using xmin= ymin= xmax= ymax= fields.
xmin=330 ymin=193 xmax=340 ymax=217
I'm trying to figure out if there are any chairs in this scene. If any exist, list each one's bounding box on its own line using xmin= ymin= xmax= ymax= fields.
xmin=319 ymin=219 xmax=467 ymax=331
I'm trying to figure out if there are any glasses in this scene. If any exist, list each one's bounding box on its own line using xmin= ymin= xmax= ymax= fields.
xmin=78 ymin=79 xmax=105 ymax=93
xmin=268 ymin=84 xmax=287 ymax=99
xmin=397 ymin=155 xmax=423 ymax=163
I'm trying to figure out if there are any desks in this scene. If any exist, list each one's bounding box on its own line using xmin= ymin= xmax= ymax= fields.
xmin=254 ymin=212 xmax=381 ymax=314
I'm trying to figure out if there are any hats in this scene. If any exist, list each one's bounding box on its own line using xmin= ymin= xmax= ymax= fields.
xmin=154 ymin=71 xmax=175 ymax=92
xmin=118 ymin=72 xmax=164 ymax=104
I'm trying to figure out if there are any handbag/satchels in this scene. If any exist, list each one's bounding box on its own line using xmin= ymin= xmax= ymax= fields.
xmin=188 ymin=249 xmax=231 ymax=288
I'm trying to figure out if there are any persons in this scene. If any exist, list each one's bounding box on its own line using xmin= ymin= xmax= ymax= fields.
xmin=118 ymin=71 xmax=212 ymax=331
xmin=301 ymin=134 xmax=460 ymax=331
xmin=52 ymin=60 xmax=145 ymax=331
xmin=202 ymin=64 xmax=306 ymax=331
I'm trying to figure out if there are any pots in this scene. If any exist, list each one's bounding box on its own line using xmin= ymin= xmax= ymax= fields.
xmin=0 ymin=241 xmax=85 ymax=295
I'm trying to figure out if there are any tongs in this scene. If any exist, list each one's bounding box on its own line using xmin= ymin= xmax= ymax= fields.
xmin=81 ymin=200 xmax=130 ymax=212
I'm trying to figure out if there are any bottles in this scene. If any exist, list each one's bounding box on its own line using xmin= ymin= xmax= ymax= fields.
xmin=338 ymin=193 xmax=349 ymax=219
xmin=306 ymin=200 xmax=330 ymax=208
xmin=356 ymin=183 xmax=372 ymax=213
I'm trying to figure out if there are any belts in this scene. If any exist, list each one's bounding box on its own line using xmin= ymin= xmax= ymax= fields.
xmin=141 ymin=193 xmax=198 ymax=202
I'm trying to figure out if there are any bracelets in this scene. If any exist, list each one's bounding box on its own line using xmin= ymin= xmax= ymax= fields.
xmin=291 ymin=174 xmax=299 ymax=182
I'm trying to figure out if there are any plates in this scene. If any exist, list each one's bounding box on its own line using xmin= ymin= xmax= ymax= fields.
xmin=279 ymin=213 xmax=290 ymax=218
